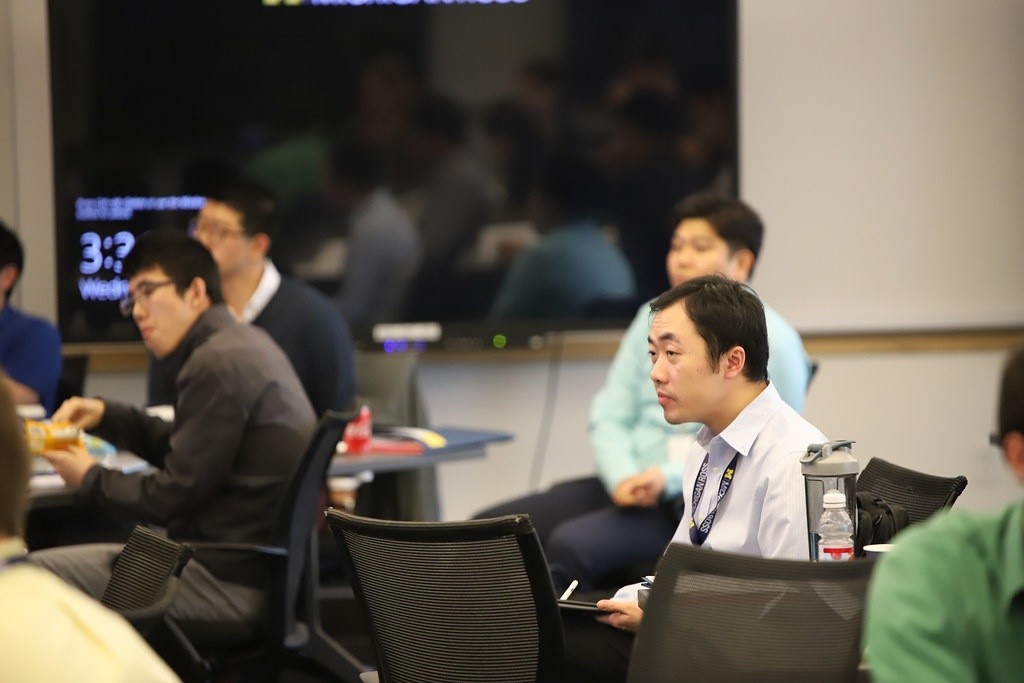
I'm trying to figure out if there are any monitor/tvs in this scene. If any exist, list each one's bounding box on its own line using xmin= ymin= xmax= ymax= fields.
xmin=47 ymin=0 xmax=741 ymax=345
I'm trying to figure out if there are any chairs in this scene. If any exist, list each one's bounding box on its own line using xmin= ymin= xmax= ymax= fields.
xmin=101 ymin=345 xmax=427 ymax=683
xmin=853 ymin=457 xmax=968 ymax=522
xmin=626 ymin=542 xmax=884 ymax=683
xmin=326 ymin=506 xmax=574 ymax=683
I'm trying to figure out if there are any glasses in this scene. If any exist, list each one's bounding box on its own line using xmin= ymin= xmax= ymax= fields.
xmin=188 ymin=218 xmax=254 ymax=246
xmin=120 ymin=280 xmax=178 ymax=318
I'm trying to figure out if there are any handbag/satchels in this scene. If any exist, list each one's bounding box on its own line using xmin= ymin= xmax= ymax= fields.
xmin=855 ymin=490 xmax=909 ymax=558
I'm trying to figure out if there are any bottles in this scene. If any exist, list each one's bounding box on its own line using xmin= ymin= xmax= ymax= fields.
xmin=818 ymin=489 xmax=855 ymax=562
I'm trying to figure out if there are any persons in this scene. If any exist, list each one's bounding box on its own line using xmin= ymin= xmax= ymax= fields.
xmin=554 ymin=274 xmax=841 ymax=682
xmin=545 ymin=186 xmax=810 ymax=593
xmin=0 ymin=378 xmax=184 ymax=683
xmin=858 ymin=346 xmax=1023 ymax=683
xmin=140 ymin=176 xmax=359 ymax=417
xmin=262 ymin=48 xmax=732 ymax=330
xmin=0 ymin=218 xmax=61 ymax=418
xmin=25 ymin=230 xmax=316 ymax=616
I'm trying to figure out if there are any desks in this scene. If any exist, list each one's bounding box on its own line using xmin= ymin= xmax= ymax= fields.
xmin=22 ymin=425 xmax=516 ymax=640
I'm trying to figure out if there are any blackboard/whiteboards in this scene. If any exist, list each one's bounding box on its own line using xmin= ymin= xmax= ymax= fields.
xmin=0 ymin=0 xmax=1024 ymax=385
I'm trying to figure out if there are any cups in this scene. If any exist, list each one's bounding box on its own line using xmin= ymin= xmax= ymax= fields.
xmin=799 ymin=438 xmax=859 ymax=562
xmin=862 ymin=543 xmax=896 ymax=559
xmin=344 ymin=405 xmax=372 ymax=453
